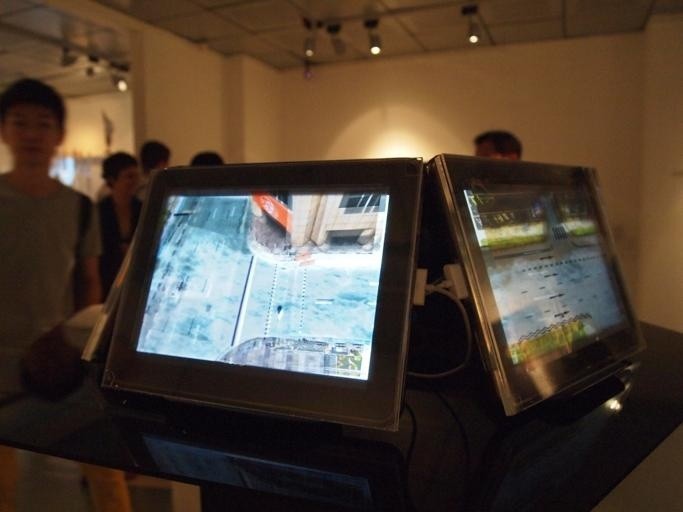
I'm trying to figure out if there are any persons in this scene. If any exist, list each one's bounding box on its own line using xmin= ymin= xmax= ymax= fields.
xmin=1 ymin=77 xmax=135 ymax=511
xmin=189 ymin=150 xmax=225 ymax=165
xmin=473 ymin=128 xmax=522 ymax=159
xmin=95 ymin=148 xmax=144 ymax=304
xmin=134 ymin=138 xmax=170 ymax=203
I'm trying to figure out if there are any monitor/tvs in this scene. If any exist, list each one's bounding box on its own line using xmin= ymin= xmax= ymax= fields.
xmin=101 ymin=156 xmax=425 ymax=435
xmin=427 ymin=152 xmax=650 ymax=418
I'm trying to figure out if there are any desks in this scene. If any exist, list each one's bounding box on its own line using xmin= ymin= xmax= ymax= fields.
xmin=1 ymin=317 xmax=683 ymax=511
